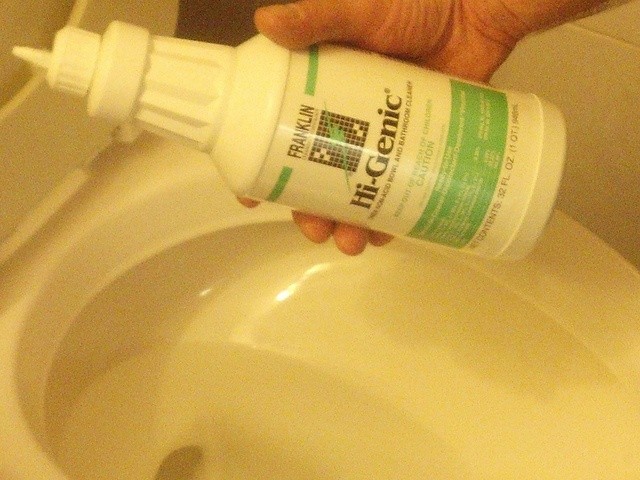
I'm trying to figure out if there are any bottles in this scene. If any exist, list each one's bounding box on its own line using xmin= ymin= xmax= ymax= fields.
xmin=12 ymin=19 xmax=568 ymax=260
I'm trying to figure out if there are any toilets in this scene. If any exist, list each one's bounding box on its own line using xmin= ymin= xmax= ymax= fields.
xmin=0 ymin=0 xmax=639 ymax=479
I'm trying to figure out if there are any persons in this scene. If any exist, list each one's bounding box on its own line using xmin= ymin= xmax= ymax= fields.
xmin=231 ymin=0 xmax=627 ymax=257
